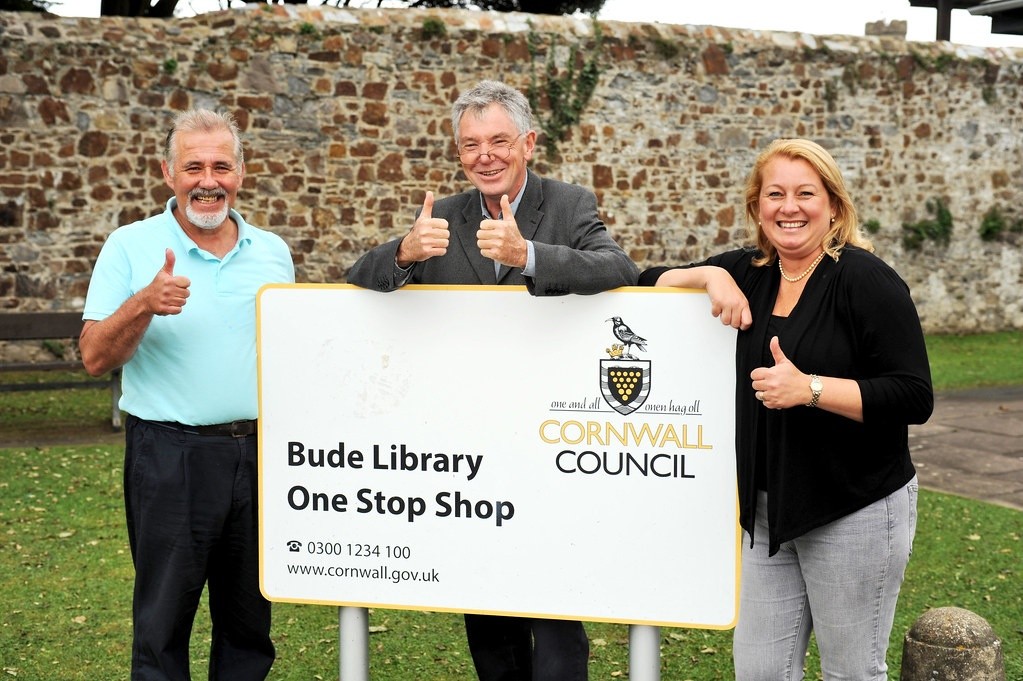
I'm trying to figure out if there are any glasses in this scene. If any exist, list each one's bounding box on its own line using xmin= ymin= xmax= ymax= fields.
xmin=456 ymin=134 xmax=522 ymax=164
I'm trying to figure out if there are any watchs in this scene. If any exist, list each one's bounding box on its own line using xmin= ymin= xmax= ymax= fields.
xmin=806 ymin=374 xmax=823 ymax=409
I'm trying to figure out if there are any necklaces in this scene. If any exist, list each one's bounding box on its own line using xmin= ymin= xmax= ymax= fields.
xmin=777 ymin=233 xmax=836 ymax=282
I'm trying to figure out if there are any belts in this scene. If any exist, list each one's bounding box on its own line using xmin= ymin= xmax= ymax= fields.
xmin=150 ymin=419 xmax=258 ymax=437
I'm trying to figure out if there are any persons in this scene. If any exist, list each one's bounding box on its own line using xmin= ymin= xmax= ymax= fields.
xmin=636 ymin=136 xmax=935 ymax=681
xmin=79 ymin=109 xmax=297 ymax=681
xmin=349 ymin=81 xmax=644 ymax=679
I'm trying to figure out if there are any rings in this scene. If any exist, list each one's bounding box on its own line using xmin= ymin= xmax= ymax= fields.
xmin=759 ymin=390 xmax=764 ymax=400
